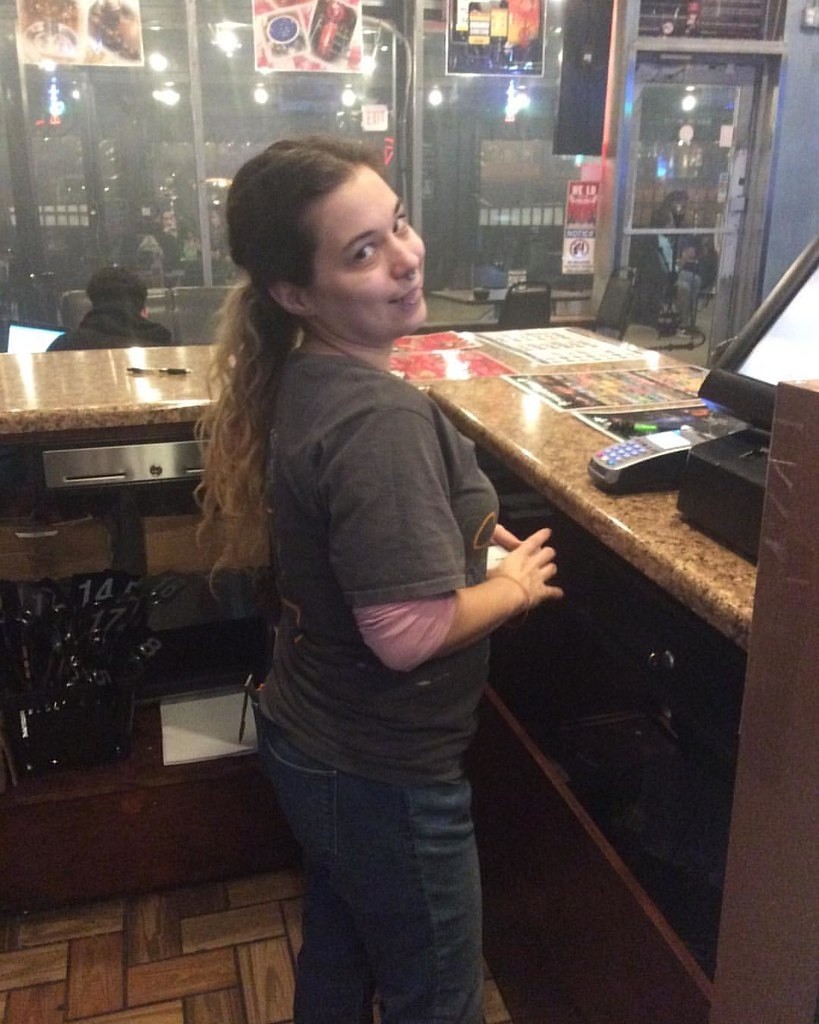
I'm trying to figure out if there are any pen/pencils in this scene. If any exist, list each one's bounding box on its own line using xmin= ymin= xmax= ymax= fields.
xmin=609 ymin=421 xmax=680 ymax=432
xmin=127 ymin=366 xmax=192 ymax=373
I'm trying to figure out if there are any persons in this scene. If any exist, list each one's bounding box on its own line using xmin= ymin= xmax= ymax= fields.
xmin=648 ymin=192 xmax=720 ymax=339
xmin=200 ymin=136 xmax=563 ymax=1023
xmin=46 ymin=267 xmax=170 ymax=353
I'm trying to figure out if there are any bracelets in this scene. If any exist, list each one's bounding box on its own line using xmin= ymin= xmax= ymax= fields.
xmin=486 ymin=574 xmax=529 ymax=629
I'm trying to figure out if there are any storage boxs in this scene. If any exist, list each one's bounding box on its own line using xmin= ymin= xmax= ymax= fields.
xmin=140 ymin=515 xmax=270 ymax=579
xmin=0 ymin=518 xmax=114 ymax=581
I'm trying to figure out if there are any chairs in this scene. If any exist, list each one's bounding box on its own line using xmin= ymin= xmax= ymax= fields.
xmin=496 ymin=280 xmax=550 ymax=332
xmin=593 ymin=265 xmax=640 ymax=338
xmin=61 ymin=285 xmax=232 ymax=341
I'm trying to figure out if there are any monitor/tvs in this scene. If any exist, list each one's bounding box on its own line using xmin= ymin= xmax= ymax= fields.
xmin=697 ymin=232 xmax=819 ymax=430
